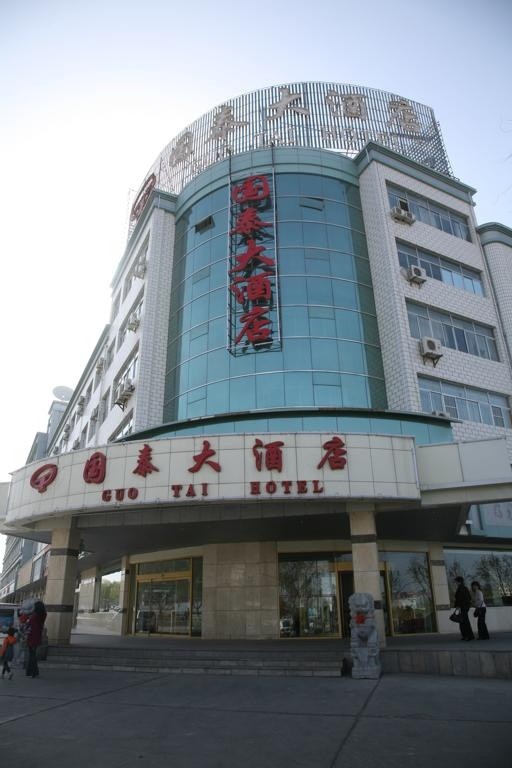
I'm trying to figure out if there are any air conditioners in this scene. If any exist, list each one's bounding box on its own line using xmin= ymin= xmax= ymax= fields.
xmin=391 ymin=207 xmax=406 ymax=219
xmin=52 ymin=255 xmax=148 ymax=456
xmin=403 ymin=211 xmax=416 ymax=225
xmin=420 ymin=336 xmax=442 ymax=359
xmin=432 ymin=411 xmax=451 ymax=418
xmin=408 ymin=265 xmax=426 ymax=283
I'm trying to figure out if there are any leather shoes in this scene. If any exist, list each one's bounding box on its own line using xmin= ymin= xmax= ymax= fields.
xmin=461 ymin=634 xmax=475 ymax=641
xmin=477 ymin=635 xmax=490 ymax=640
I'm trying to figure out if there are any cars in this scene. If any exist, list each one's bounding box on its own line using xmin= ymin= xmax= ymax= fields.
xmin=0 ymin=601 xmax=23 ymax=659
xmin=279 ymin=618 xmax=291 ymax=636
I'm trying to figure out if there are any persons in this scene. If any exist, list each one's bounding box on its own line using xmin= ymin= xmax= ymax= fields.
xmin=23 ymin=601 xmax=48 ymax=677
xmin=471 ymin=580 xmax=490 ymax=641
xmin=453 ymin=575 xmax=475 ymax=640
xmin=1 ymin=627 xmax=17 ymax=680
xmin=12 ymin=613 xmax=28 ymax=672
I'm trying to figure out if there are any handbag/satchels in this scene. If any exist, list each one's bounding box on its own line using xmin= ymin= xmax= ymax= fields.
xmin=474 ymin=608 xmax=480 ymax=617
xmin=449 ymin=607 xmax=464 ymax=623
